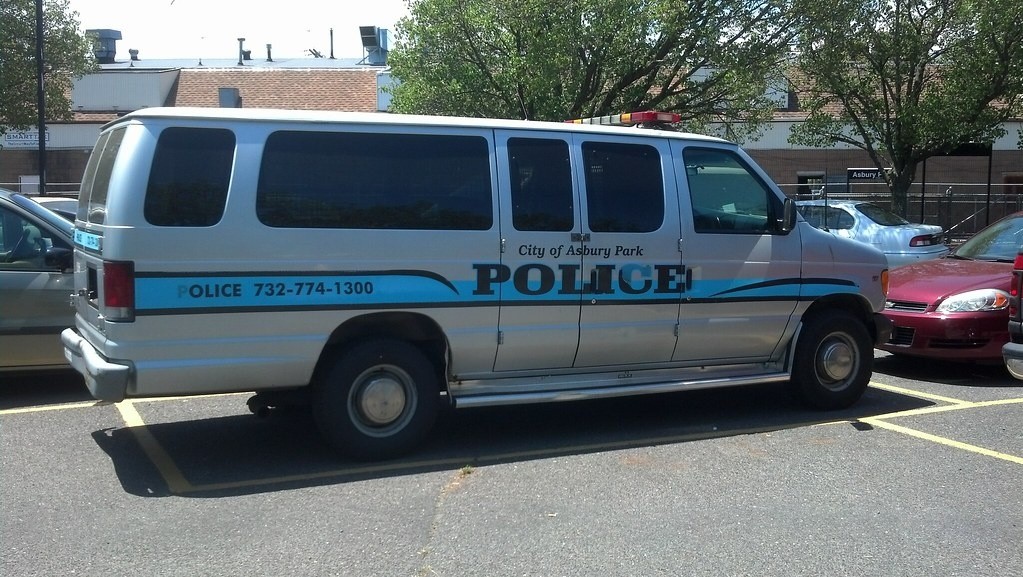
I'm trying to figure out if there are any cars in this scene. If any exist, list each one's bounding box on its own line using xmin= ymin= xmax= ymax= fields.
xmin=0 ymin=187 xmax=86 ymax=393
xmin=20 ymin=195 xmax=81 ymax=251
xmin=874 ymin=210 xmax=1023 ymax=367
xmin=793 ymin=197 xmax=950 ymax=268
xmin=1001 ymin=239 xmax=1023 ymax=380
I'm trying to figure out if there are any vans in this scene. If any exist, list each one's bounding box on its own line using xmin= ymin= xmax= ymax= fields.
xmin=61 ymin=109 xmax=894 ymax=468
